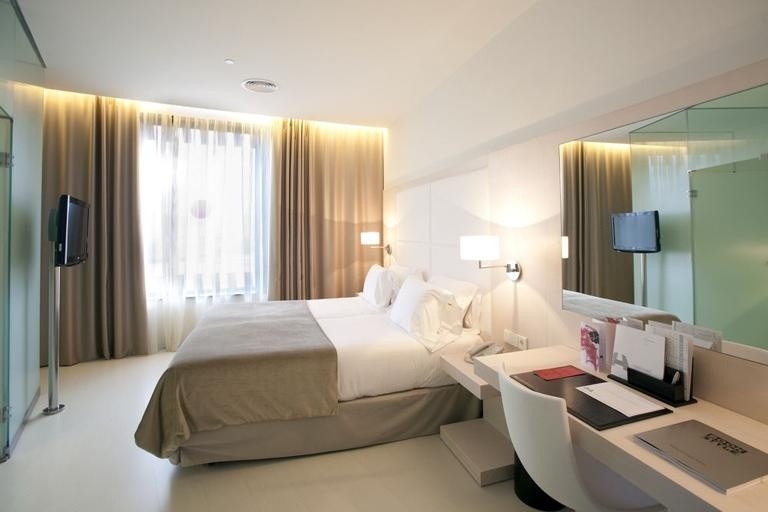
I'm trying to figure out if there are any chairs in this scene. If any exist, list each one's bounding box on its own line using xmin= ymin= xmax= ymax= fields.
xmin=498 ymin=361 xmax=666 ymax=512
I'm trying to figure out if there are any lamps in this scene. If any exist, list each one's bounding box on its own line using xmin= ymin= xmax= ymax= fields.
xmin=360 ymin=231 xmax=392 ymax=254
xmin=458 ymin=233 xmax=521 ymax=281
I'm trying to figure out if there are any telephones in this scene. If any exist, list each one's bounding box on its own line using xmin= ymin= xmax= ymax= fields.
xmin=468 ymin=341 xmax=504 ymax=362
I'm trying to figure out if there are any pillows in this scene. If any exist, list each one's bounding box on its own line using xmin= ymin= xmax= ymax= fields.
xmin=360 ymin=263 xmax=483 ymax=352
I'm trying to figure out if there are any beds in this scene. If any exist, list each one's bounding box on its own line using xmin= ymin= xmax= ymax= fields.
xmin=168 ymin=241 xmax=492 ymax=466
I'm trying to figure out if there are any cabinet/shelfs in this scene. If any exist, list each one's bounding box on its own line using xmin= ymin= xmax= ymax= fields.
xmin=440 ymin=352 xmax=517 ymax=487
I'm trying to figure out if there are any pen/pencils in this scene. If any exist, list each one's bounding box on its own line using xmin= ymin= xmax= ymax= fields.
xmin=671 ymin=371 xmax=680 ymax=384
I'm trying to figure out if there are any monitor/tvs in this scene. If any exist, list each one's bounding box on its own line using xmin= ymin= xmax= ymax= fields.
xmin=609 ymin=209 xmax=662 ymax=255
xmin=54 ymin=193 xmax=91 ymax=268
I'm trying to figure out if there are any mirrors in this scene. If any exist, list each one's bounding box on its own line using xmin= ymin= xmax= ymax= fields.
xmin=560 ymin=84 xmax=767 ymax=365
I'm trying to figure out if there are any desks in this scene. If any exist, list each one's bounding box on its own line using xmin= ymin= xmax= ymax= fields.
xmin=471 ymin=348 xmax=768 ymax=512
